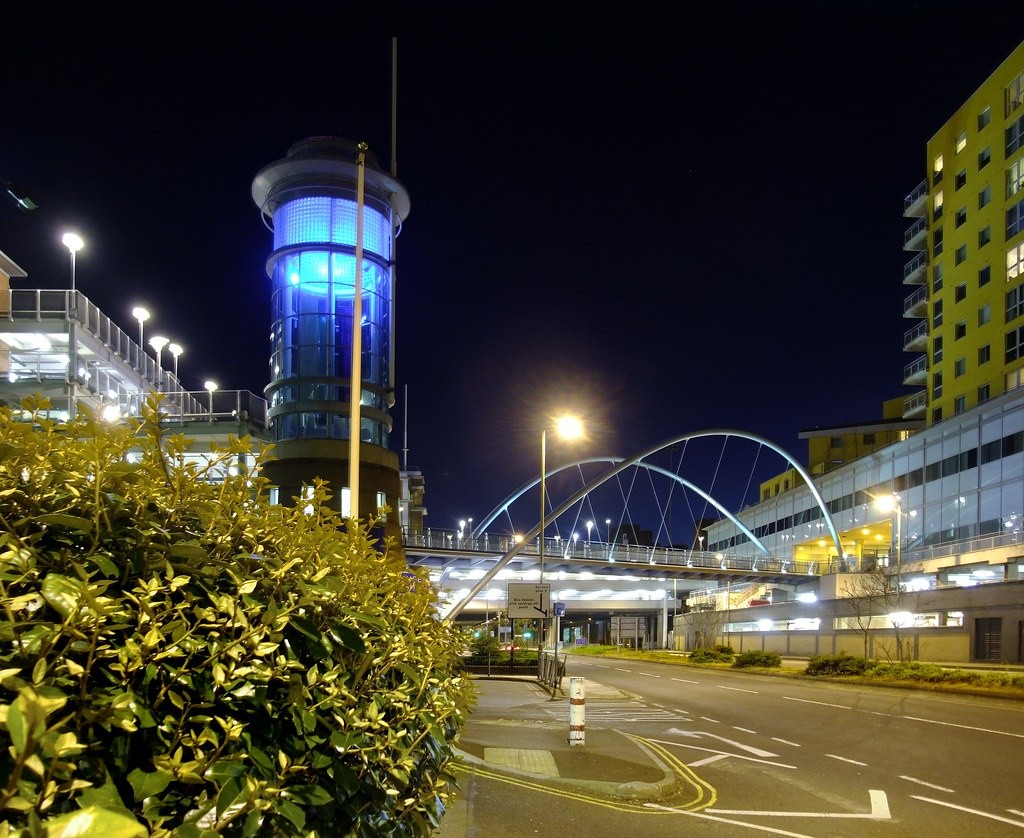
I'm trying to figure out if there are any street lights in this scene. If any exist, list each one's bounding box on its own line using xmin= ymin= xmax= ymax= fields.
xmin=537 ymin=414 xmax=586 ymax=683
xmin=875 ymin=497 xmax=901 ymax=662
